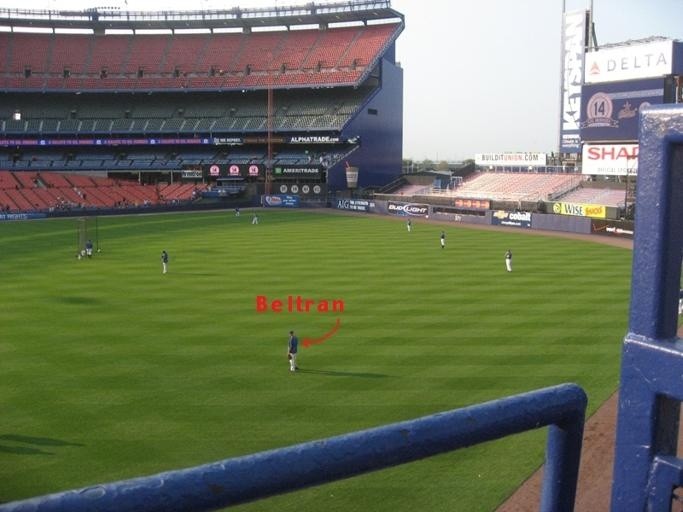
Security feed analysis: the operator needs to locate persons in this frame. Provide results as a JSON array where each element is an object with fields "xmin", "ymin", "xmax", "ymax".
[
  {"xmin": 678, "ymin": 287, "xmax": 683, "ymax": 315},
  {"xmin": 234, "ymin": 207, "xmax": 239, "ymax": 217},
  {"xmin": 439, "ymin": 230, "xmax": 446, "ymax": 248},
  {"xmin": 404, "ymin": 218, "xmax": 412, "ymax": 231},
  {"xmin": 286, "ymin": 328, "xmax": 300, "ymax": 373},
  {"xmin": 502, "ymin": 248, "xmax": 514, "ymax": 273},
  {"xmin": 161, "ymin": 249, "xmax": 169, "ymax": 274},
  {"xmin": 85, "ymin": 238, "xmax": 94, "ymax": 258},
  {"xmin": 251, "ymin": 213, "xmax": 259, "ymax": 225}
]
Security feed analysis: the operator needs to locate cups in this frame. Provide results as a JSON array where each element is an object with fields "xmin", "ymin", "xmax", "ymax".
[{"xmin": 345, "ymin": 166, "xmax": 360, "ymax": 190}]
